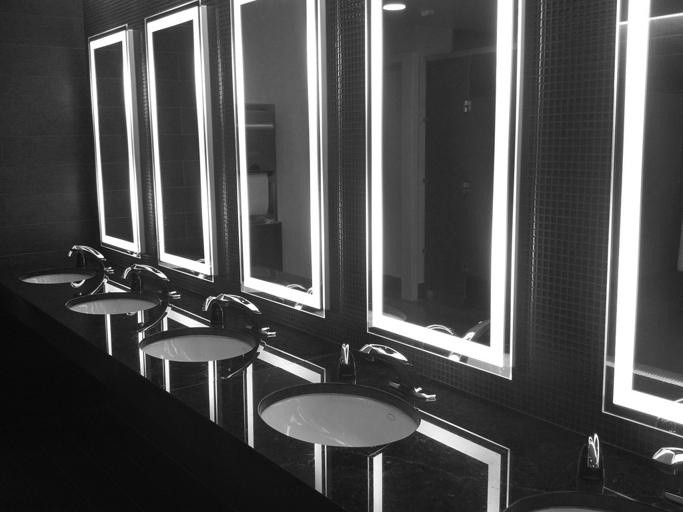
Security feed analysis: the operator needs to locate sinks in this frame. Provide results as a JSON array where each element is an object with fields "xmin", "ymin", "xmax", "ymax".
[
  {"xmin": 136, "ymin": 322, "xmax": 262, "ymax": 369},
  {"xmin": 16, "ymin": 267, "xmax": 97, "ymax": 286},
  {"xmin": 61, "ymin": 290, "xmax": 168, "ymax": 320},
  {"xmin": 504, "ymin": 489, "xmax": 660, "ymax": 512},
  {"xmin": 254, "ymin": 373, "xmax": 422, "ymax": 452}
]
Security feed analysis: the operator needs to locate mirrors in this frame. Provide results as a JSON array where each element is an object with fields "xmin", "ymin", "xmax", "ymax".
[
  {"xmin": 366, "ymin": 3, "xmax": 525, "ymax": 385},
  {"xmin": 146, "ymin": 0, "xmax": 214, "ymax": 284},
  {"xmin": 601, "ymin": 1, "xmax": 683, "ymax": 436},
  {"xmin": 88, "ymin": 21, "xmax": 142, "ymax": 260},
  {"xmin": 233, "ymin": 1, "xmax": 325, "ymax": 312}
]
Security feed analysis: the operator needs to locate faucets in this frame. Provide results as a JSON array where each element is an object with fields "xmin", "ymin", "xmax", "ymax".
[
  {"xmin": 65, "ymin": 243, "xmax": 107, "ymax": 281},
  {"xmin": 460, "ymin": 318, "xmax": 487, "ymax": 342},
  {"xmin": 119, "ymin": 260, "xmax": 172, "ymax": 297},
  {"xmin": 356, "ymin": 340, "xmax": 436, "ymax": 394},
  {"xmin": 650, "ymin": 445, "xmax": 683, "ymax": 510},
  {"xmin": 287, "ymin": 282, "xmax": 313, "ymax": 294},
  {"xmin": 202, "ymin": 292, "xmax": 263, "ymax": 329}
]
